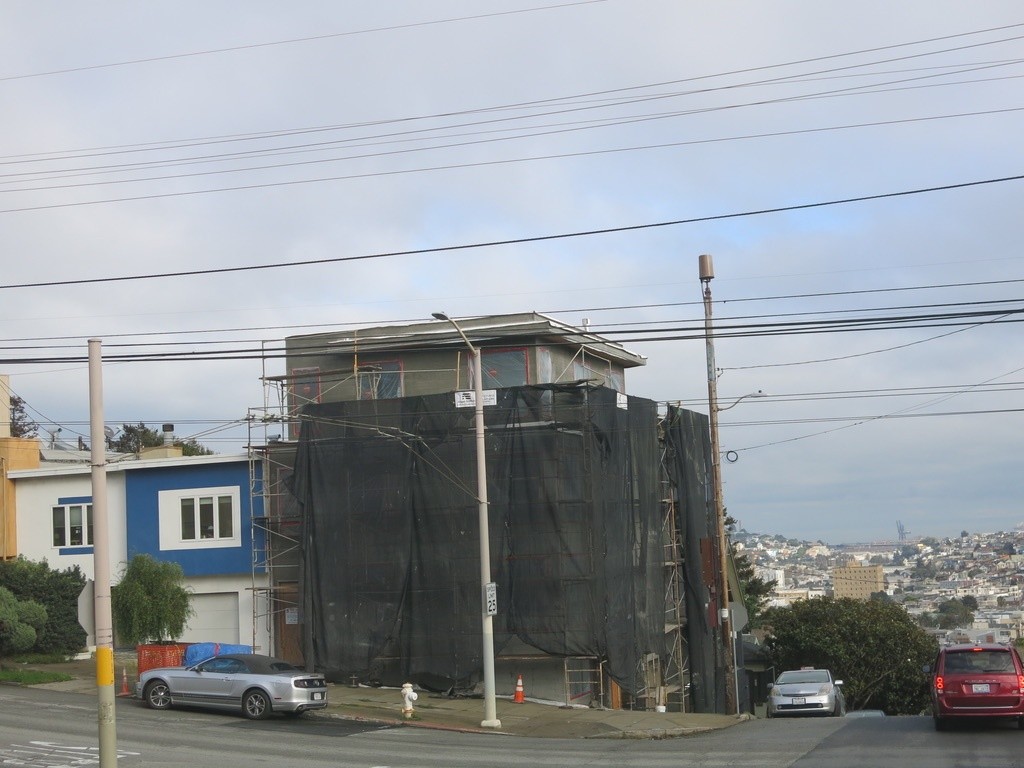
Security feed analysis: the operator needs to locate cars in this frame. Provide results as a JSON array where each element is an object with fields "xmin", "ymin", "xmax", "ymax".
[
  {"xmin": 845, "ymin": 711, "xmax": 885, "ymax": 717},
  {"xmin": 136, "ymin": 653, "xmax": 327, "ymax": 721},
  {"xmin": 766, "ymin": 669, "xmax": 844, "ymax": 717}
]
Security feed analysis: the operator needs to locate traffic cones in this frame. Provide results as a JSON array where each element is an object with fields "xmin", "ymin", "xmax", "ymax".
[
  {"xmin": 512, "ymin": 675, "xmax": 525, "ymax": 703},
  {"xmin": 116, "ymin": 667, "xmax": 130, "ymax": 696}
]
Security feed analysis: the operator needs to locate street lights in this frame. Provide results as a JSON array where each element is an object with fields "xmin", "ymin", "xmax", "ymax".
[
  {"xmin": 430, "ymin": 312, "xmax": 501, "ymax": 729},
  {"xmin": 708, "ymin": 392, "xmax": 767, "ymax": 715}
]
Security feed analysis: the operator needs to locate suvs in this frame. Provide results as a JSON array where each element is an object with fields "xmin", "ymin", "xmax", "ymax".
[{"xmin": 922, "ymin": 643, "xmax": 1024, "ymax": 732}]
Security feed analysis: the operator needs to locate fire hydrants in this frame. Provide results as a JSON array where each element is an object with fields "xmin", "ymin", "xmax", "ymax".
[{"xmin": 401, "ymin": 683, "xmax": 418, "ymax": 721}]
